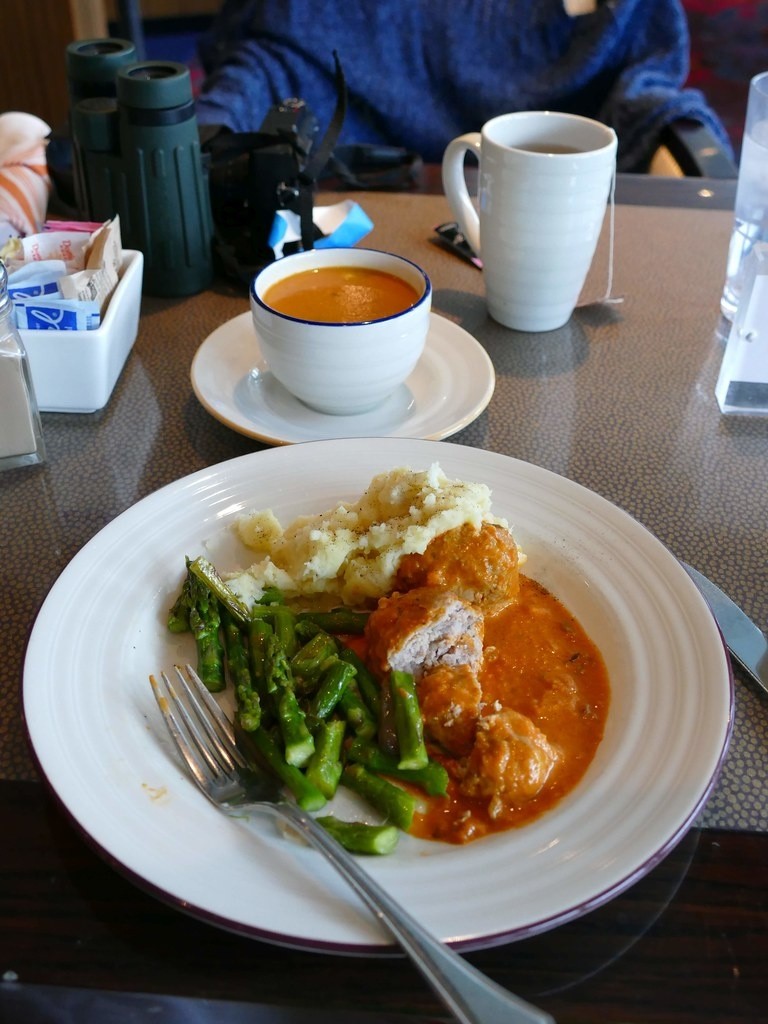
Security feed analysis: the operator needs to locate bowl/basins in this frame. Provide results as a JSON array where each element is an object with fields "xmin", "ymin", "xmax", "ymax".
[{"xmin": 7, "ymin": 249, "xmax": 145, "ymax": 418}]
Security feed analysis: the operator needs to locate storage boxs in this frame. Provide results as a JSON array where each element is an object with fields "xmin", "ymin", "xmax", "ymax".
[{"xmin": 17, "ymin": 250, "xmax": 144, "ymax": 413}]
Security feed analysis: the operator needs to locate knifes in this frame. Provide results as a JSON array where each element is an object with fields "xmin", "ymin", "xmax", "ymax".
[{"xmin": 673, "ymin": 554, "xmax": 768, "ymax": 693}]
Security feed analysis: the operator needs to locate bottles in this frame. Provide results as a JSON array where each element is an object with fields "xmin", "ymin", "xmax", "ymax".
[{"xmin": 0, "ymin": 260, "xmax": 47, "ymax": 470}]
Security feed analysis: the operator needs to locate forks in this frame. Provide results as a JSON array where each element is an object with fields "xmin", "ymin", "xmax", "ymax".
[{"xmin": 148, "ymin": 664, "xmax": 558, "ymax": 1024}]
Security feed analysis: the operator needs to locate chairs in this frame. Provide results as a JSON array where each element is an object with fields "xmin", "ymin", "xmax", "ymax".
[{"xmin": 195, "ymin": 0, "xmax": 738, "ymax": 179}]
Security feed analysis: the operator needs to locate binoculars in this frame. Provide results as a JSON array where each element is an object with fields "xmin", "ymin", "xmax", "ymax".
[{"xmin": 65, "ymin": 38, "xmax": 214, "ymax": 298}]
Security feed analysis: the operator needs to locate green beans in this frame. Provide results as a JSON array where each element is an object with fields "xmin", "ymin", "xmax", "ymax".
[{"xmin": 167, "ymin": 557, "xmax": 449, "ymax": 853}]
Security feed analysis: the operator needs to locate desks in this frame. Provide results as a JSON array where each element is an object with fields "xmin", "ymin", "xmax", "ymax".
[{"xmin": 0, "ymin": 159, "xmax": 768, "ymax": 1024}]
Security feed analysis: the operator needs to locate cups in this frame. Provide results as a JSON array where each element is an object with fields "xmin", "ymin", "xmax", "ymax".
[
  {"xmin": 247, "ymin": 247, "xmax": 433, "ymax": 416},
  {"xmin": 111, "ymin": 61, "xmax": 219, "ymax": 296},
  {"xmin": 442, "ymin": 111, "xmax": 617, "ymax": 334},
  {"xmin": 713, "ymin": 71, "xmax": 768, "ymax": 322},
  {"xmin": 64, "ymin": 37, "xmax": 140, "ymax": 225}
]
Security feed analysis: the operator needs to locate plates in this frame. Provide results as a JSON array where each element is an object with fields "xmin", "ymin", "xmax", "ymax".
[
  {"xmin": 21, "ymin": 439, "xmax": 738, "ymax": 962},
  {"xmin": 189, "ymin": 308, "xmax": 496, "ymax": 444}
]
